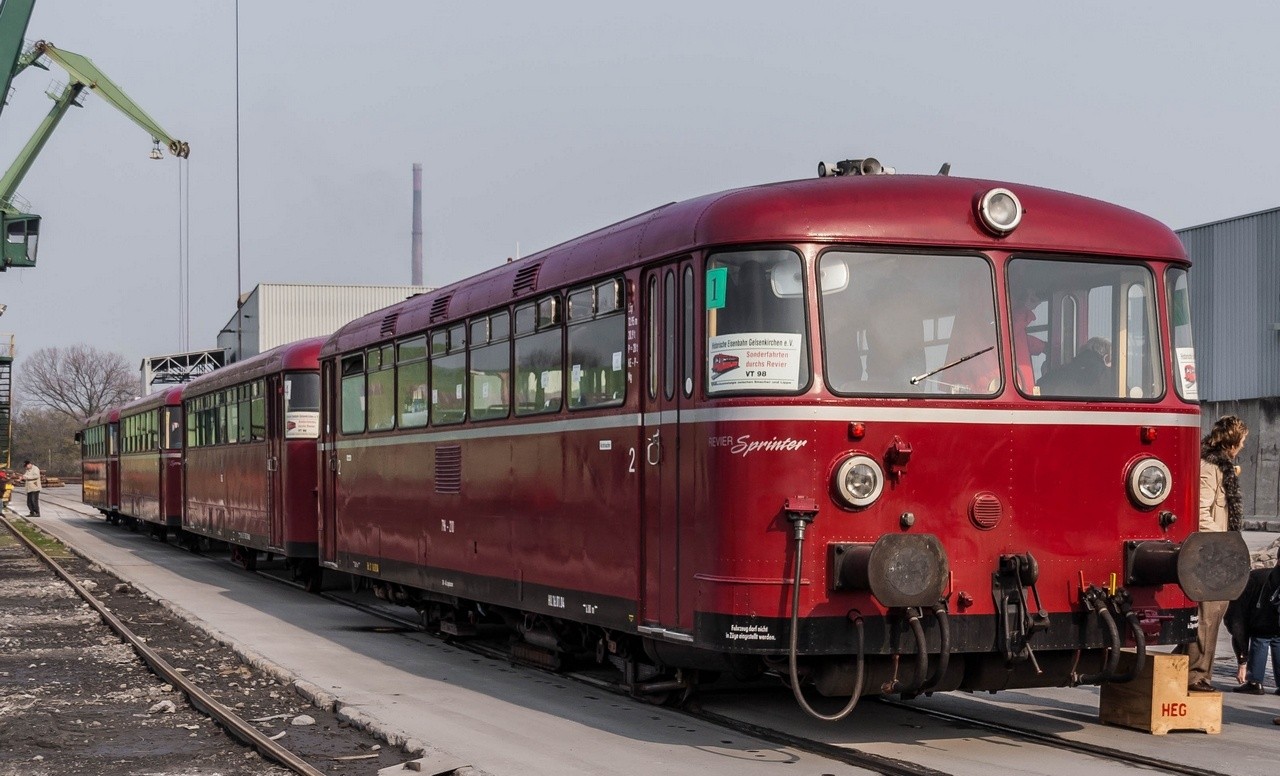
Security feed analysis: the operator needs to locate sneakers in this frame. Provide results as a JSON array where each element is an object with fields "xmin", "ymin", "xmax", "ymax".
[
  {"xmin": 1234, "ymin": 681, "xmax": 1265, "ymax": 695},
  {"xmin": 1275, "ymin": 688, "xmax": 1280, "ymax": 696}
]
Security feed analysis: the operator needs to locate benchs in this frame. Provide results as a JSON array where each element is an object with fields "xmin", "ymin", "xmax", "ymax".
[{"xmin": 483, "ymin": 391, "xmax": 621, "ymax": 417}]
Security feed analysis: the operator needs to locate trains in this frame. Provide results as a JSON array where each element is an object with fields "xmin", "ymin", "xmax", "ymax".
[{"xmin": 75, "ymin": 157, "xmax": 1253, "ymax": 725}]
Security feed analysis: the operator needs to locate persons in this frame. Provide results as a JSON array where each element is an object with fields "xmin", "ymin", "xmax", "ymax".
[
  {"xmin": 17, "ymin": 460, "xmax": 42, "ymax": 517},
  {"xmin": 1181, "ymin": 414, "xmax": 1279, "ymax": 695},
  {"xmin": 839, "ymin": 261, "xmax": 1131, "ymax": 401}
]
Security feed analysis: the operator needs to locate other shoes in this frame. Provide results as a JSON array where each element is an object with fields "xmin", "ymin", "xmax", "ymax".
[
  {"xmin": 27, "ymin": 513, "xmax": 34, "ymax": 516},
  {"xmin": 1273, "ymin": 717, "xmax": 1280, "ymax": 726},
  {"xmin": 33, "ymin": 513, "xmax": 40, "ymax": 517}
]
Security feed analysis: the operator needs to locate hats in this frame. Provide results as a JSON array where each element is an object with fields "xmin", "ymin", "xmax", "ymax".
[{"xmin": 23, "ymin": 461, "xmax": 30, "ymax": 468}]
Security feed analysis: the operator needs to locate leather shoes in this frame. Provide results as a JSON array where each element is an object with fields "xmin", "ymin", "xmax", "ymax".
[{"xmin": 1190, "ymin": 680, "xmax": 1215, "ymax": 692}]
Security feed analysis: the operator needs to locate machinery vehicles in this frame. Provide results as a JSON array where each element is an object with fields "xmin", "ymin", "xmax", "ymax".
[{"xmin": 0, "ymin": 1, "xmax": 190, "ymax": 300}]
